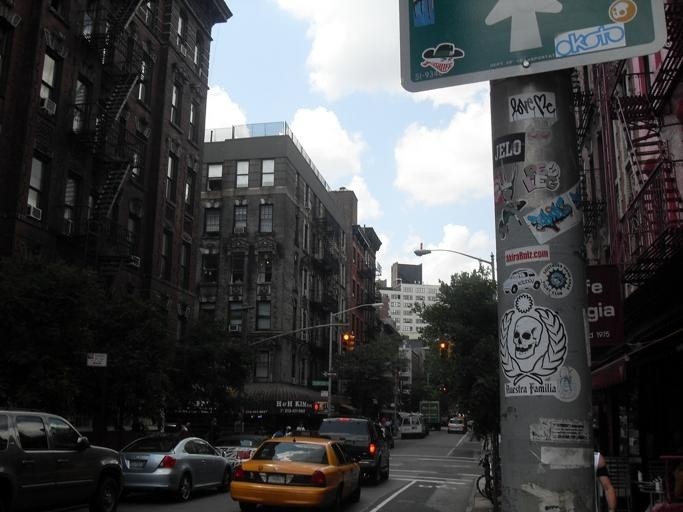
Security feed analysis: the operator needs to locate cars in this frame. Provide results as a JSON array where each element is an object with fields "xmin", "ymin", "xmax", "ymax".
[
  {"xmin": 318, "ymin": 418, "xmax": 389, "ymax": 486},
  {"xmin": 214, "ymin": 435, "xmax": 267, "ymax": 469},
  {"xmin": 120, "ymin": 436, "xmax": 232, "ymax": 500},
  {"xmin": 448, "ymin": 417, "xmax": 466, "ymax": 434},
  {"xmin": 230, "ymin": 436, "xmax": 360, "ymax": 512}
]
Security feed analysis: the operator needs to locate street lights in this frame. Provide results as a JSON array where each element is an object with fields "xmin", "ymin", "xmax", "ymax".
[
  {"xmin": 414, "ymin": 250, "xmax": 496, "ymax": 301},
  {"xmin": 328, "ymin": 303, "xmax": 384, "ymax": 418}
]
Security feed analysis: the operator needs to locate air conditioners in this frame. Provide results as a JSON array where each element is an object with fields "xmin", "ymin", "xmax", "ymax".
[
  {"xmin": 228, "ymin": 324, "xmax": 242, "ymax": 333},
  {"xmin": 234, "ymin": 225, "xmax": 246, "ymax": 235},
  {"xmin": 26, "ymin": 205, "xmax": 42, "ymax": 221},
  {"xmin": 129, "ymin": 255, "xmax": 141, "ymax": 268},
  {"xmin": 40, "ymin": 97, "xmax": 57, "ymax": 115}
]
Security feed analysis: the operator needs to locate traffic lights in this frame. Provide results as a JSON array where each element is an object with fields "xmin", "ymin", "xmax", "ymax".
[
  {"xmin": 342, "ymin": 329, "xmax": 357, "ymax": 352},
  {"xmin": 440, "ymin": 341, "xmax": 449, "ymax": 360}
]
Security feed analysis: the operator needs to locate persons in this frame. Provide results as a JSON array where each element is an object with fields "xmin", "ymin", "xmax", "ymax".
[
  {"xmin": 273, "ymin": 424, "xmax": 283, "ymax": 437},
  {"xmin": 284, "ymin": 425, "xmax": 293, "ymax": 436},
  {"xmin": 297, "ymin": 421, "xmax": 305, "ymax": 431},
  {"xmin": 177, "ymin": 422, "xmax": 193, "ymax": 431},
  {"xmin": 594, "ymin": 451, "xmax": 616, "ymax": 512}
]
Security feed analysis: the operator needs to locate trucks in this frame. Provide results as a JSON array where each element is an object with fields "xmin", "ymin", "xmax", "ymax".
[{"xmin": 419, "ymin": 400, "xmax": 441, "ymax": 431}]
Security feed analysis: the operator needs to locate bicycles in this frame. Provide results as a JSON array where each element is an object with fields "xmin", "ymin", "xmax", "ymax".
[{"xmin": 477, "ymin": 439, "xmax": 494, "ymax": 505}]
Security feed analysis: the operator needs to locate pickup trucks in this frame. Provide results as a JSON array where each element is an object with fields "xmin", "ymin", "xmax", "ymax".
[{"xmin": 399, "ymin": 415, "xmax": 426, "ymax": 439}]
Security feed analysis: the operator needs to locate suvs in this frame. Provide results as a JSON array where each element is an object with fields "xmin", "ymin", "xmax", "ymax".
[{"xmin": 0, "ymin": 411, "xmax": 124, "ymax": 512}]
